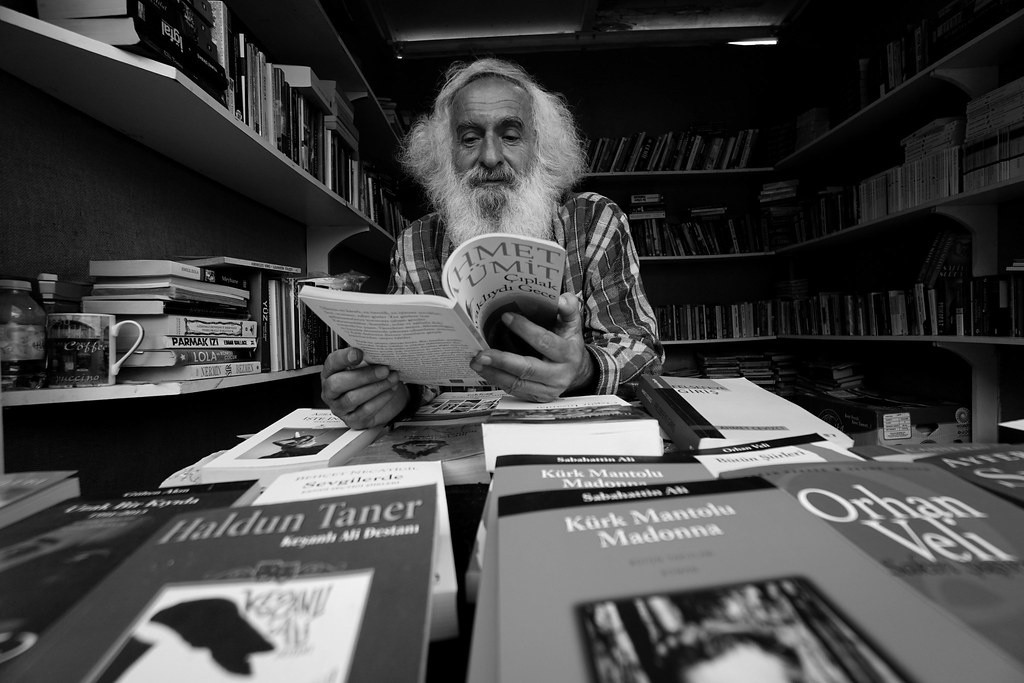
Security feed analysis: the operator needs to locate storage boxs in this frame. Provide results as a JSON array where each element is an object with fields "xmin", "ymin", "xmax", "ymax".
[{"xmin": 793, "ymin": 389, "xmax": 971, "ymax": 446}]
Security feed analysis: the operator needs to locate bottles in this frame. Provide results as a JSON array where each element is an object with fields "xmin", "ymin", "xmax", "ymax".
[{"xmin": 0, "ymin": 279, "xmax": 49, "ymax": 390}]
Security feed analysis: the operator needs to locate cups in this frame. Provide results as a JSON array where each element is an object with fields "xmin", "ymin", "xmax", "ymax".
[{"xmin": 43, "ymin": 311, "xmax": 144, "ymax": 390}]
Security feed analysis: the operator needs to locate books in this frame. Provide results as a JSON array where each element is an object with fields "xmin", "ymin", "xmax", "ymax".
[
  {"xmin": 0, "ymin": 377, "xmax": 1024, "ymax": 683},
  {"xmin": 650, "ymin": 298, "xmax": 772, "ymax": 341},
  {"xmin": 770, "ymin": 352, "xmax": 863, "ymax": 396},
  {"xmin": 770, "ymin": 232, "xmax": 1024, "ymax": 338},
  {"xmin": 299, "ymin": 230, "xmax": 569, "ymax": 386},
  {"xmin": 757, "ymin": 75, "xmax": 1024, "ymax": 242},
  {"xmin": 580, "ymin": 124, "xmax": 757, "ymax": 173},
  {"xmin": 623, "ymin": 192, "xmax": 765, "ymax": 255},
  {"xmin": 37, "ymin": 0, "xmax": 415, "ymax": 243},
  {"xmin": 35, "ymin": 254, "xmax": 365, "ymax": 377},
  {"xmin": 661, "ymin": 348, "xmax": 777, "ymax": 385},
  {"xmin": 793, "ymin": 0, "xmax": 1024, "ymax": 151}
]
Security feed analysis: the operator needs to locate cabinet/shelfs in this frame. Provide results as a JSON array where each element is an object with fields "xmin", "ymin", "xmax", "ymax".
[
  {"xmin": 579, "ymin": 168, "xmax": 778, "ymax": 345},
  {"xmin": 0, "ymin": 1, "xmax": 405, "ymax": 404},
  {"xmin": 777, "ymin": 7, "xmax": 1023, "ymax": 449}
]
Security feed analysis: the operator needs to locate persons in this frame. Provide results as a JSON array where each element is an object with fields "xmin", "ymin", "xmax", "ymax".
[{"xmin": 320, "ymin": 59, "xmax": 668, "ymax": 429}]
